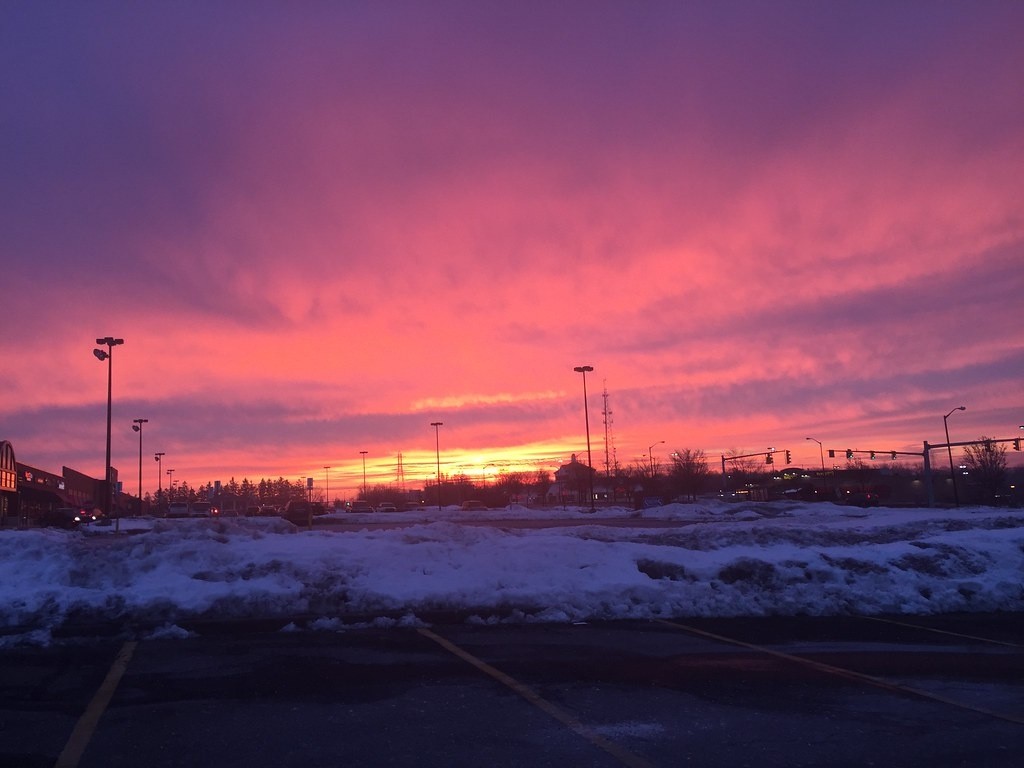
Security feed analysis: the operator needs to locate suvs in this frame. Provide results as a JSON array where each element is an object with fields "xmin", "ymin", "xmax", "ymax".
[{"xmin": 281, "ymin": 499, "xmax": 313, "ymax": 524}]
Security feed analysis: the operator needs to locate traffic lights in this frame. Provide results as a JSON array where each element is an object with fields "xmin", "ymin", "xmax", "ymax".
[
  {"xmin": 984, "ymin": 441, "xmax": 990, "ymax": 452},
  {"xmin": 786, "ymin": 450, "xmax": 791, "ymax": 464},
  {"xmin": 892, "ymin": 452, "xmax": 897, "ymax": 460},
  {"xmin": 871, "ymin": 451, "xmax": 875, "ymax": 460},
  {"xmin": 1013, "ymin": 440, "xmax": 1019, "ymax": 451},
  {"xmin": 768, "ymin": 453, "xmax": 773, "ymax": 464},
  {"xmin": 847, "ymin": 449, "xmax": 853, "ymax": 458}
]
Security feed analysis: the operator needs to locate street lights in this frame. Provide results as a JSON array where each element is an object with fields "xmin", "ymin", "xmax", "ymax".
[
  {"xmin": 649, "ymin": 441, "xmax": 665, "ymax": 477},
  {"xmin": 167, "ymin": 470, "xmax": 175, "ymax": 488},
  {"xmin": 360, "ymin": 452, "xmax": 368, "ymax": 493},
  {"xmin": 324, "ymin": 467, "xmax": 330, "ymax": 508},
  {"xmin": 574, "ymin": 366, "xmax": 594, "ymax": 509},
  {"xmin": 132, "ymin": 419, "xmax": 149, "ymax": 516},
  {"xmin": 806, "ymin": 438, "xmax": 825, "ymax": 485},
  {"xmin": 94, "ymin": 337, "xmax": 124, "ymax": 518},
  {"xmin": 943, "ymin": 406, "xmax": 966, "ymax": 507},
  {"xmin": 154, "ymin": 453, "xmax": 165, "ymax": 489},
  {"xmin": 432, "ymin": 423, "xmax": 443, "ymax": 511}
]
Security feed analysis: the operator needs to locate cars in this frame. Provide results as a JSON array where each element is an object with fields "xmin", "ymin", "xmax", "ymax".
[
  {"xmin": 39, "ymin": 507, "xmax": 105, "ymax": 531},
  {"xmin": 845, "ymin": 492, "xmax": 879, "ymax": 509},
  {"xmin": 166, "ymin": 502, "xmax": 397, "ymax": 518},
  {"xmin": 462, "ymin": 500, "xmax": 488, "ymax": 511}
]
